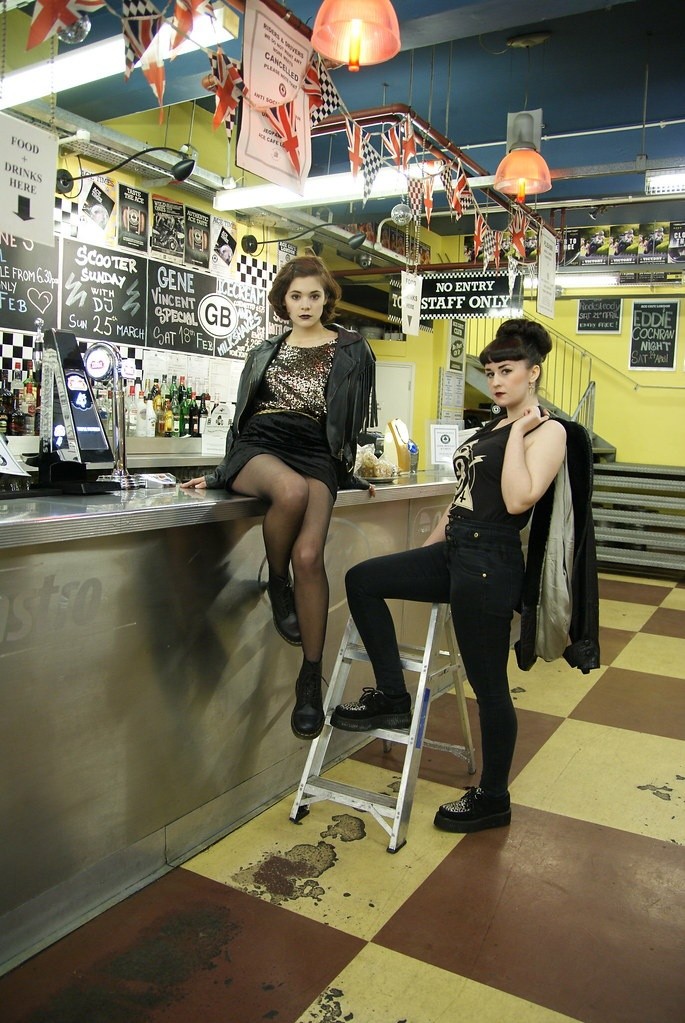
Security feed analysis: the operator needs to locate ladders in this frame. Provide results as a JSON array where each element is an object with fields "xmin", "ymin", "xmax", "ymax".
[{"xmin": 288, "ymin": 579, "xmax": 478, "ymax": 854}]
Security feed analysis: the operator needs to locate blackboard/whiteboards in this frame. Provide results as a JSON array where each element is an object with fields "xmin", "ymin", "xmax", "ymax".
[
  {"xmin": 1, "ymin": 231, "xmax": 59, "ymax": 335},
  {"xmin": 628, "ymin": 300, "xmax": 681, "ymax": 371},
  {"xmin": 216, "ymin": 276, "xmax": 267, "ymax": 361},
  {"xmin": 58, "ymin": 233, "xmax": 147, "ymax": 349},
  {"xmin": 268, "ymin": 301, "xmax": 293, "ymax": 339},
  {"xmin": 576, "ymin": 298, "xmax": 623, "ymax": 335},
  {"xmin": 148, "ymin": 256, "xmax": 218, "ymax": 359}
]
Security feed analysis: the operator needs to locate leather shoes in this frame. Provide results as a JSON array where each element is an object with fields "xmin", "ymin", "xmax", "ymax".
[
  {"xmin": 330, "ymin": 686, "xmax": 413, "ymax": 732},
  {"xmin": 434, "ymin": 785, "xmax": 512, "ymax": 832}
]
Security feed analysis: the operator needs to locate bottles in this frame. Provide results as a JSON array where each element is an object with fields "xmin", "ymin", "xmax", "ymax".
[
  {"xmin": 0, "ymin": 359, "xmax": 42, "ymax": 437},
  {"xmin": 116, "ymin": 369, "xmax": 220, "ymax": 438}
]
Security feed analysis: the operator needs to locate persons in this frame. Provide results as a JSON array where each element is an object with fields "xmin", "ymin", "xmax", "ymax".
[
  {"xmin": 179, "ymin": 253, "xmax": 379, "ymax": 739},
  {"xmin": 330, "ymin": 318, "xmax": 567, "ymax": 833}
]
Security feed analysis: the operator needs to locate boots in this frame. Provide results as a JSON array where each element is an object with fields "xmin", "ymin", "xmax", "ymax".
[
  {"xmin": 265, "ymin": 567, "xmax": 303, "ymax": 645},
  {"xmin": 289, "ymin": 655, "xmax": 331, "ymax": 740}
]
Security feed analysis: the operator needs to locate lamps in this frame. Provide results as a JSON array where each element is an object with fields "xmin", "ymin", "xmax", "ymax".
[
  {"xmin": 213, "ymin": 159, "xmax": 443, "ymax": 211},
  {"xmin": 311, "ymin": 0, "xmax": 402, "ymax": 72},
  {"xmin": 242, "ymin": 223, "xmax": 367, "ymax": 254},
  {"xmin": 0, "ymin": 0, "xmax": 240, "ymax": 111},
  {"xmin": 493, "ymin": 31, "xmax": 552, "ymax": 203},
  {"xmin": 588, "ymin": 204, "xmax": 606, "ymax": 221},
  {"xmin": 56, "ymin": 147, "xmax": 195, "ymax": 194},
  {"xmin": 374, "ymin": 204, "xmax": 412, "ymax": 251}
]
